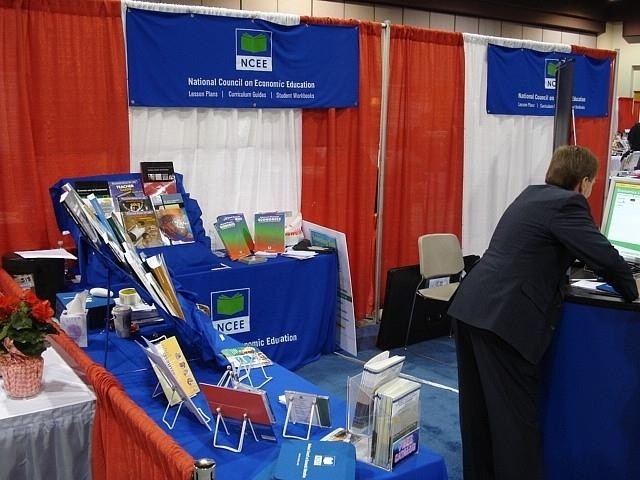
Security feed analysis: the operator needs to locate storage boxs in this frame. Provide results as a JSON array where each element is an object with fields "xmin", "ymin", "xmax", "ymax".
[{"xmin": 62, "ymin": 309, "xmax": 88, "ymax": 348}]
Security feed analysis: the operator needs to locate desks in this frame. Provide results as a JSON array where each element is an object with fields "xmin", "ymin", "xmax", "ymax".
[
  {"xmin": 40, "ymin": 281, "xmax": 446, "ymax": 480},
  {"xmin": 109, "ymin": 242, "xmax": 339, "ymax": 370}
]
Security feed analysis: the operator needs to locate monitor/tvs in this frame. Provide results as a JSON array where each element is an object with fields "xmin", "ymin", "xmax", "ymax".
[{"xmin": 600, "ymin": 175, "xmax": 640, "ymax": 266}]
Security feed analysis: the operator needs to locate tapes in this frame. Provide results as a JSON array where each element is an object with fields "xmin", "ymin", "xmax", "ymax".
[{"xmin": 118, "ymin": 287, "xmax": 140, "ymax": 304}]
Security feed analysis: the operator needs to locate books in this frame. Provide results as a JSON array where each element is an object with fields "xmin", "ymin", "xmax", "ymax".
[
  {"xmin": 351, "ymin": 350, "xmax": 421, "ymax": 470},
  {"xmin": 60, "ymin": 162, "xmax": 194, "ymax": 322},
  {"xmin": 213, "ymin": 211, "xmax": 285, "ymax": 261}
]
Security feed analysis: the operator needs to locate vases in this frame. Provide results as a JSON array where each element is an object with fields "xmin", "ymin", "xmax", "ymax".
[{"xmin": 1, "ymin": 355, "xmax": 44, "ymax": 401}]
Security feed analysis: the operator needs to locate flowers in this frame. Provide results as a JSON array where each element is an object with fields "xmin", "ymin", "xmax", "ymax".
[{"xmin": 0, "ymin": 289, "xmax": 61, "ymax": 355}]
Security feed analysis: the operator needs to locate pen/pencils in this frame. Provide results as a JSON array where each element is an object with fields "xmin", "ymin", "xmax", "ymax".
[{"xmin": 568, "ymin": 278, "xmax": 600, "ymax": 281}]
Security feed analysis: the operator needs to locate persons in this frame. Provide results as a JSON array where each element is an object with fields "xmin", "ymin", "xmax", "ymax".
[
  {"xmin": 445, "ymin": 146, "xmax": 639, "ymax": 480},
  {"xmin": 620, "ymin": 122, "xmax": 640, "ymax": 170}
]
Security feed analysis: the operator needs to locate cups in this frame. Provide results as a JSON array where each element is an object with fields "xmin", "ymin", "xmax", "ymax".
[{"xmin": 112, "ymin": 305, "xmax": 133, "ymax": 339}]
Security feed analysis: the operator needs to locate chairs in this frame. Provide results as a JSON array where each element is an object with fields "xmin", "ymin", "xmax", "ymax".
[{"xmin": 404, "ymin": 234, "xmax": 465, "ymax": 353}]
